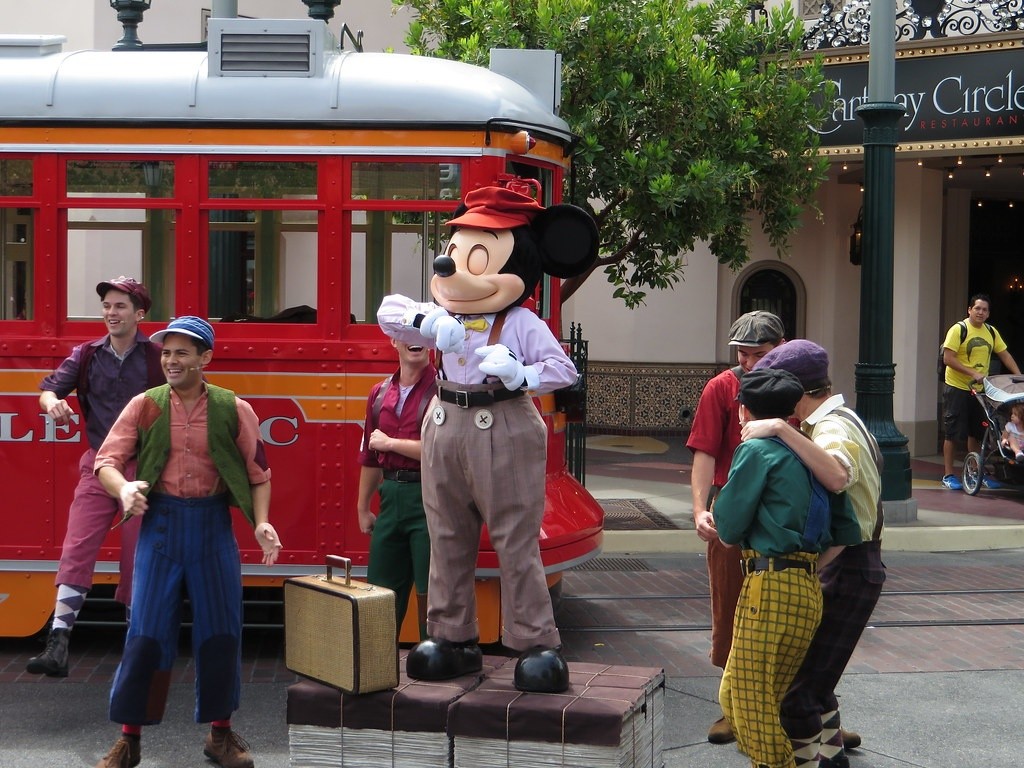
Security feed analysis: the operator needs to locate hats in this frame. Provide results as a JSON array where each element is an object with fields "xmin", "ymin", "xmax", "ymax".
[
  {"xmin": 728, "ymin": 311, "xmax": 783, "ymax": 346},
  {"xmin": 754, "ymin": 340, "xmax": 829, "ymax": 381},
  {"xmin": 148, "ymin": 315, "xmax": 215, "ymax": 350},
  {"xmin": 734, "ymin": 370, "xmax": 802, "ymax": 417},
  {"xmin": 96, "ymin": 276, "xmax": 151, "ymax": 313}
]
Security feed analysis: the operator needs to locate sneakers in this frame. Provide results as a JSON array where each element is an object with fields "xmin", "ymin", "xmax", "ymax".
[
  {"xmin": 95, "ymin": 736, "xmax": 140, "ymax": 768},
  {"xmin": 971, "ymin": 476, "xmax": 1001, "ymax": 489},
  {"xmin": 942, "ymin": 477, "xmax": 962, "ymax": 490},
  {"xmin": 204, "ymin": 729, "xmax": 254, "ymax": 768}
]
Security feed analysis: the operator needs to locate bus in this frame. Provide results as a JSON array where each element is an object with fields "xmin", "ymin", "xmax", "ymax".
[{"xmin": 0, "ymin": 0, "xmax": 607, "ymax": 643}]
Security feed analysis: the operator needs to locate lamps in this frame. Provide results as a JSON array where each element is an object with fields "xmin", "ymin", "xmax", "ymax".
[{"xmin": 850, "ymin": 206, "xmax": 864, "ymax": 265}]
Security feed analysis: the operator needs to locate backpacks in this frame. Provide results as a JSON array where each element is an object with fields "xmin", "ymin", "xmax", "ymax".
[{"xmin": 937, "ymin": 320, "xmax": 994, "ymax": 379}]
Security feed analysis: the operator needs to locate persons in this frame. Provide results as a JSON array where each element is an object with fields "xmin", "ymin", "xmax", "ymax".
[
  {"xmin": 1001, "ymin": 402, "xmax": 1024, "ymax": 460},
  {"xmin": 357, "ymin": 337, "xmax": 438, "ymax": 643},
  {"xmin": 713, "ymin": 366, "xmax": 863, "ymax": 768},
  {"xmin": 941, "ymin": 295, "xmax": 1021, "ymax": 489},
  {"xmin": 686, "ymin": 311, "xmax": 861, "ymax": 748},
  {"xmin": 92, "ymin": 316, "xmax": 281, "ymax": 768},
  {"xmin": 740, "ymin": 340, "xmax": 887, "ymax": 768},
  {"xmin": 25, "ymin": 277, "xmax": 167, "ymax": 678}
]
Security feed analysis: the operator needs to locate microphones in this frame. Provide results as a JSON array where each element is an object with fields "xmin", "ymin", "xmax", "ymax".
[
  {"xmin": 120, "ymin": 320, "xmax": 127, "ymax": 324},
  {"xmin": 189, "ymin": 365, "xmax": 208, "ymax": 371}
]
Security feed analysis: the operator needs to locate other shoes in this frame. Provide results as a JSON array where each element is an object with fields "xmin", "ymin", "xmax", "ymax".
[
  {"xmin": 842, "ymin": 728, "xmax": 860, "ymax": 747},
  {"xmin": 708, "ymin": 716, "xmax": 736, "ymax": 742}
]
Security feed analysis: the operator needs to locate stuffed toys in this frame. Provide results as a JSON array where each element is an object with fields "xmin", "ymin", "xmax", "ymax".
[{"xmin": 375, "ymin": 187, "xmax": 600, "ymax": 694}]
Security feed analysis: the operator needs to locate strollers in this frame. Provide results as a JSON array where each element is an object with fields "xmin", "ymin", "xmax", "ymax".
[{"xmin": 961, "ymin": 374, "xmax": 1024, "ymax": 499}]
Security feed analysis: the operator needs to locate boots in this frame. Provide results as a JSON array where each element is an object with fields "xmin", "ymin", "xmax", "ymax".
[{"xmin": 27, "ymin": 630, "xmax": 69, "ymax": 678}]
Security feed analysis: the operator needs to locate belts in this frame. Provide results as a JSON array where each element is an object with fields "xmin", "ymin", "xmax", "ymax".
[
  {"xmin": 738, "ymin": 558, "xmax": 811, "ymax": 576},
  {"xmin": 436, "ymin": 385, "xmax": 526, "ymax": 408},
  {"xmin": 382, "ymin": 469, "xmax": 423, "ymax": 485}
]
